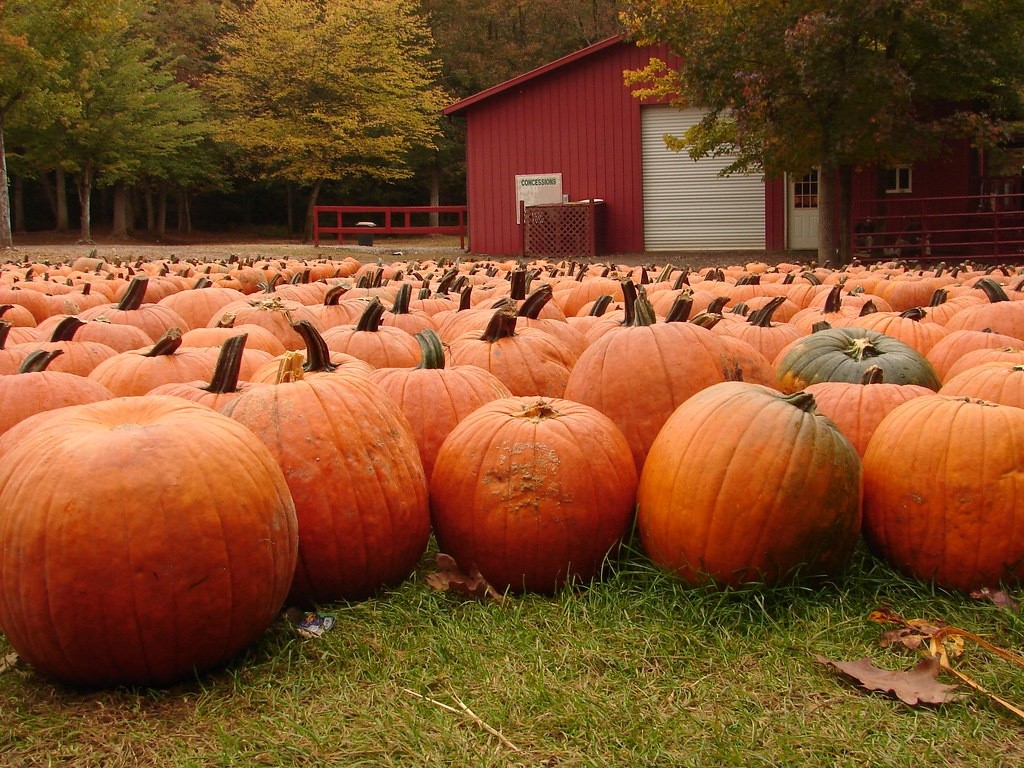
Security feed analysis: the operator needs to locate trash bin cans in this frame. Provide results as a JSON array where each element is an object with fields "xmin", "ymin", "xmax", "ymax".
[{"xmin": 356, "ymin": 221, "xmax": 377, "ymax": 247}]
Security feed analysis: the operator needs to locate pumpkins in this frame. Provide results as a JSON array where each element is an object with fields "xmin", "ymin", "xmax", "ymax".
[{"xmin": 0, "ymin": 249, "xmax": 1024, "ymax": 688}]
